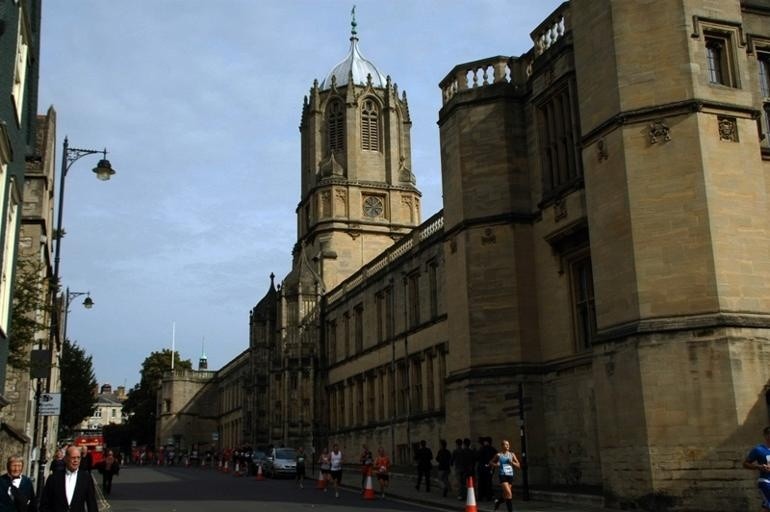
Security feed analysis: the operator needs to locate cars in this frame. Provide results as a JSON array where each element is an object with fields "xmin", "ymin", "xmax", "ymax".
[
  {"xmin": 57, "ymin": 438, "xmax": 74, "ymax": 447},
  {"xmin": 243, "ymin": 446, "xmax": 297, "ymax": 477}
]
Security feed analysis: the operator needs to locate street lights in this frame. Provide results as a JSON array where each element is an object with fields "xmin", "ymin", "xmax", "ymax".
[
  {"xmin": 55, "ymin": 285, "xmax": 95, "ymax": 453},
  {"xmin": 37, "ymin": 134, "xmax": 117, "ymax": 506}
]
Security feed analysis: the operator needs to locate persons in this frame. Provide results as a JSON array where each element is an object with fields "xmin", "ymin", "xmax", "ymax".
[
  {"xmin": 0, "ymin": 443, "xmax": 125, "ymax": 512},
  {"xmin": 128, "ymin": 443, "xmax": 213, "ymax": 469},
  {"xmin": 293, "ymin": 436, "xmax": 522, "ymax": 510}
]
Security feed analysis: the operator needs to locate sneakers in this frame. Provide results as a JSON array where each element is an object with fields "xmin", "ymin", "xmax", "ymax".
[{"xmin": 298, "ymin": 484, "xmax": 388, "ymax": 500}]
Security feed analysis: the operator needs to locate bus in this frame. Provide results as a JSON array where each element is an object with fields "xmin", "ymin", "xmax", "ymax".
[{"xmin": 76, "ymin": 433, "xmax": 105, "ymax": 467}]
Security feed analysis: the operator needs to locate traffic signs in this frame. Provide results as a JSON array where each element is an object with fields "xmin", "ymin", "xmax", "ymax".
[{"xmin": 501, "ymin": 391, "xmax": 533, "ymax": 418}]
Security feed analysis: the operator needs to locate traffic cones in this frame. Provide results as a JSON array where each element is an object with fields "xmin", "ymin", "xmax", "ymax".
[
  {"xmin": 217, "ymin": 457, "xmax": 223, "ymax": 471},
  {"xmin": 256, "ymin": 461, "xmax": 264, "ymax": 482},
  {"xmin": 185, "ymin": 458, "xmax": 189, "ymax": 468},
  {"xmin": 223, "ymin": 459, "xmax": 231, "ymax": 473},
  {"xmin": 463, "ymin": 475, "xmax": 477, "ymax": 512},
  {"xmin": 232, "ymin": 461, "xmax": 242, "ymax": 478},
  {"xmin": 315, "ymin": 470, "xmax": 324, "ymax": 489},
  {"xmin": 362, "ymin": 468, "xmax": 375, "ymax": 501}
]
